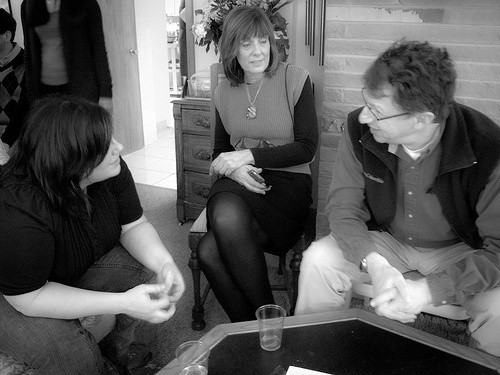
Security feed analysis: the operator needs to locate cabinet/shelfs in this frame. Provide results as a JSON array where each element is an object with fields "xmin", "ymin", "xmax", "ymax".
[{"xmin": 170, "ymin": 97, "xmax": 213, "ymax": 224}]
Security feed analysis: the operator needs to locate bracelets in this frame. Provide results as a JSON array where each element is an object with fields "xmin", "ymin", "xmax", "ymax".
[{"xmin": 360, "ymin": 259, "xmax": 367, "ymax": 273}]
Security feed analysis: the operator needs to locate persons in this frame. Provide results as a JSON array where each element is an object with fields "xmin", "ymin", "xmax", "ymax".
[
  {"xmin": 294, "ymin": 41, "xmax": 500, "ymax": 358},
  {"xmin": 0, "ymin": 97, "xmax": 186, "ymax": 375},
  {"xmin": 21, "ymin": 0, "xmax": 113, "ymax": 116},
  {"xmin": 196, "ymin": 5, "xmax": 319, "ymax": 322},
  {"xmin": 0, "ymin": 8, "xmax": 26, "ymax": 163}
]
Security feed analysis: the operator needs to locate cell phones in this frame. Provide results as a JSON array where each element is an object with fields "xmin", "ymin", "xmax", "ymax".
[{"xmin": 248, "ymin": 169, "xmax": 265, "ymax": 184}]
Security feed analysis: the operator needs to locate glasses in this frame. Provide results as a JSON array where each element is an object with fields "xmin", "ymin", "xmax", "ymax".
[{"xmin": 362, "ymin": 87, "xmax": 415, "ymax": 122}]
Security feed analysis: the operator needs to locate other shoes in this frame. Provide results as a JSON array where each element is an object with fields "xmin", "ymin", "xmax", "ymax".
[{"xmin": 100, "ymin": 336, "xmax": 152, "ymax": 369}]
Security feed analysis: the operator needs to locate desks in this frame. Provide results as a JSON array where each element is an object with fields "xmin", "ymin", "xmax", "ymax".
[{"xmin": 150, "ymin": 309, "xmax": 499, "ymax": 375}]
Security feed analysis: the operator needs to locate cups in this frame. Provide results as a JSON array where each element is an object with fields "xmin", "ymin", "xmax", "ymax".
[
  {"xmin": 174, "ymin": 341, "xmax": 210, "ymax": 375},
  {"xmin": 187, "ymin": 80, "xmax": 198, "ymax": 96},
  {"xmin": 255, "ymin": 303, "xmax": 286, "ymax": 351}
]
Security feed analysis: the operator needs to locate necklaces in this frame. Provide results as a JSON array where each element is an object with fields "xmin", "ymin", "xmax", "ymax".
[{"xmin": 244, "ymin": 77, "xmax": 266, "ymax": 119}]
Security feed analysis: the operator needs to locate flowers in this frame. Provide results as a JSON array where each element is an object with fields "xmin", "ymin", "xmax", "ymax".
[{"xmin": 195, "ymin": 0, "xmax": 295, "ymax": 64}]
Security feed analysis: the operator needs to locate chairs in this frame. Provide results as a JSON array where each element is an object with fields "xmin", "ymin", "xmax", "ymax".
[
  {"xmin": 351, "ymin": 271, "xmax": 471, "ymax": 322},
  {"xmin": 188, "ymin": 63, "xmax": 320, "ymax": 332}
]
